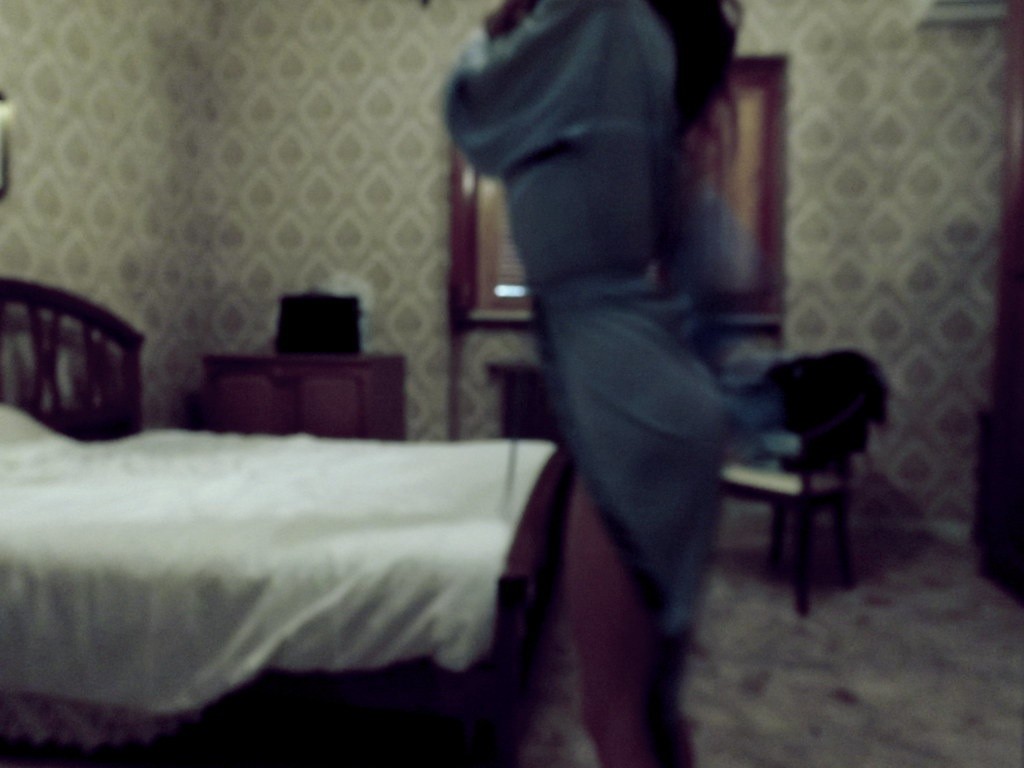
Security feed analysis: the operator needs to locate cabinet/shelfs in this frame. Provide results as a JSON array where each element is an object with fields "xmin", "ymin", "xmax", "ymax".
[{"xmin": 199, "ymin": 349, "xmax": 414, "ymax": 443}]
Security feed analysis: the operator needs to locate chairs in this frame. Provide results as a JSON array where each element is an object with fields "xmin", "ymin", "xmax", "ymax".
[{"xmin": 717, "ymin": 348, "xmax": 897, "ymax": 612}]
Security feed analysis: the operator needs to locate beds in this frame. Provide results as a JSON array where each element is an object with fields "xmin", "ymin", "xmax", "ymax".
[{"xmin": 1, "ymin": 420, "xmax": 563, "ymax": 767}]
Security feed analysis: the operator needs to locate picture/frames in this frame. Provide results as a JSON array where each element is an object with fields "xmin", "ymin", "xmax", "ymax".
[{"xmin": 441, "ymin": 52, "xmax": 793, "ymax": 337}]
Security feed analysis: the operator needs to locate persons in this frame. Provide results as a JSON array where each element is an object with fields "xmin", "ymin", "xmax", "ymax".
[{"xmin": 440, "ymin": 2, "xmax": 742, "ymax": 766}]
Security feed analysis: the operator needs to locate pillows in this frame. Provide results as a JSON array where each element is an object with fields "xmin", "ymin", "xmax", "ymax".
[{"xmin": 2, "ymin": 403, "xmax": 70, "ymax": 457}]
